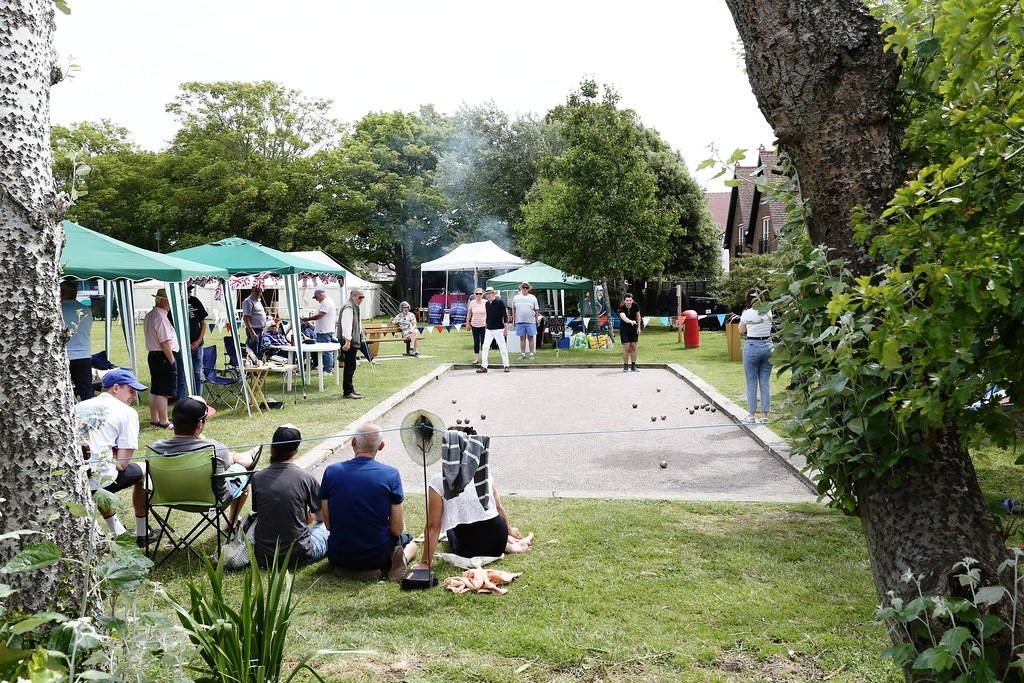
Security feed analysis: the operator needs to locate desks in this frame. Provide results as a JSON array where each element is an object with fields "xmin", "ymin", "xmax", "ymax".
[
  {"xmin": 117, "ymin": 308, "xmax": 151, "ymax": 326},
  {"xmin": 234, "ymin": 309, "xmax": 246, "ymax": 328},
  {"xmin": 236, "ymin": 368, "xmax": 272, "ymax": 417},
  {"xmin": 265, "ymin": 307, "xmax": 287, "ymax": 320},
  {"xmin": 251, "ymin": 365, "xmax": 297, "ymax": 409},
  {"xmin": 432, "ymin": 293, "xmax": 467, "ymax": 309},
  {"xmin": 282, "ymin": 343, "xmax": 341, "ymax": 392},
  {"xmin": 362, "ymin": 324, "xmax": 401, "ymax": 356}
]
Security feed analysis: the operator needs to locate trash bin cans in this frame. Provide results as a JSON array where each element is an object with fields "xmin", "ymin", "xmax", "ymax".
[
  {"xmin": 725, "ymin": 314, "xmax": 747, "ymax": 360},
  {"xmin": 682, "ymin": 309, "xmax": 700, "ymax": 349}
]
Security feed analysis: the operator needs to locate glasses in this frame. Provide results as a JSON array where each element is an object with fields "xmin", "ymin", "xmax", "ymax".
[
  {"xmin": 354, "ymin": 295, "xmax": 364, "ymax": 300},
  {"xmin": 270, "ymin": 325, "xmax": 276, "ymax": 327},
  {"xmin": 475, "ymin": 293, "xmax": 483, "ymax": 295},
  {"xmin": 522, "ymin": 287, "xmax": 529, "ymax": 290},
  {"xmin": 402, "ymin": 306, "xmax": 409, "ymax": 309},
  {"xmin": 585, "ymin": 296, "xmax": 590, "ymax": 298},
  {"xmin": 188, "ymin": 291, "xmax": 191, "ymax": 293}
]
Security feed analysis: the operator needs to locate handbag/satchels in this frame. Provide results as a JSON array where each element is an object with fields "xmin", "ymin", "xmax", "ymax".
[
  {"xmin": 213, "ymin": 513, "xmax": 258, "ymax": 569},
  {"xmin": 401, "ymin": 570, "xmax": 438, "ymax": 592}
]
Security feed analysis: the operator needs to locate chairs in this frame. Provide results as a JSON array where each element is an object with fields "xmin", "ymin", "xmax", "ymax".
[
  {"xmin": 427, "ymin": 302, "xmax": 443, "ymax": 324},
  {"xmin": 450, "ymin": 302, "xmax": 466, "ymax": 326},
  {"xmin": 145, "ymin": 445, "xmax": 262, "ymax": 575},
  {"xmin": 213, "ymin": 309, "xmax": 227, "ymax": 328},
  {"xmin": 224, "ymin": 335, "xmax": 248, "ymax": 379},
  {"xmin": 71, "ymin": 361, "xmax": 133, "ymax": 405},
  {"xmin": 202, "ymin": 345, "xmax": 247, "ymax": 411}
]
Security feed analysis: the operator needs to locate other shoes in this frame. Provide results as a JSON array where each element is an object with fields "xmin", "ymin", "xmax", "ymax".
[
  {"xmin": 318, "ymin": 371, "xmax": 332, "ymax": 376},
  {"xmin": 409, "ymin": 348, "xmax": 418, "ymax": 356},
  {"xmin": 334, "ymin": 545, "xmax": 405, "ymax": 582},
  {"xmin": 150, "ymin": 421, "xmax": 174, "ymax": 430},
  {"xmin": 342, "ymin": 392, "xmax": 362, "ymax": 399}
]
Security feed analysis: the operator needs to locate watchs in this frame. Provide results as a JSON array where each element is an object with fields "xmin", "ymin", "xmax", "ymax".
[{"xmin": 421, "ymin": 560, "xmax": 429, "ymax": 565}]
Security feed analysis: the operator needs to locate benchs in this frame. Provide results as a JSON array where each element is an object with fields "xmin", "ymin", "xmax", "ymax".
[{"xmin": 366, "ymin": 337, "xmax": 425, "ymax": 356}]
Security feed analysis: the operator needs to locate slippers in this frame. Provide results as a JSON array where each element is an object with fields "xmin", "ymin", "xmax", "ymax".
[
  {"xmin": 248, "ymin": 445, "xmax": 263, "ymax": 471},
  {"xmin": 225, "ymin": 517, "xmax": 242, "ymax": 532}
]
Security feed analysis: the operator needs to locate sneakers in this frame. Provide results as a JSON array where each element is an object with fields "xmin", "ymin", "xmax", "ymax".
[
  {"xmin": 111, "ymin": 525, "xmax": 165, "ymax": 548},
  {"xmin": 743, "ymin": 416, "xmax": 767, "ymax": 423},
  {"xmin": 623, "ymin": 363, "xmax": 639, "ymax": 372},
  {"xmin": 518, "ymin": 353, "xmax": 535, "ymax": 361},
  {"xmin": 473, "ymin": 359, "xmax": 479, "ymax": 363},
  {"xmin": 476, "ymin": 367, "xmax": 510, "ymax": 373}
]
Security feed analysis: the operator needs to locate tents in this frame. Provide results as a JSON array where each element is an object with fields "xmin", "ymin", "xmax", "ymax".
[
  {"xmin": 55, "ymin": 217, "xmax": 347, "ymax": 418},
  {"xmin": 418, "ymin": 240, "xmax": 530, "ymax": 310},
  {"xmin": 133, "ymin": 249, "xmax": 381, "ymax": 319},
  {"xmin": 486, "ymin": 260, "xmax": 600, "ymax": 350}
]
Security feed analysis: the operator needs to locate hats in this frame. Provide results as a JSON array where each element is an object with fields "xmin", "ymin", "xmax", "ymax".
[
  {"xmin": 484, "ymin": 287, "xmax": 494, "ymax": 293},
  {"xmin": 313, "ymin": 290, "xmax": 325, "ymax": 299},
  {"xmin": 519, "ymin": 281, "xmax": 532, "ymax": 290},
  {"xmin": 266, "ymin": 320, "xmax": 276, "ymax": 329},
  {"xmin": 400, "ymin": 409, "xmax": 446, "ymax": 465},
  {"xmin": 102, "ymin": 369, "xmax": 303, "ymax": 450},
  {"xmin": 151, "ymin": 285, "xmax": 195, "ymax": 300}
]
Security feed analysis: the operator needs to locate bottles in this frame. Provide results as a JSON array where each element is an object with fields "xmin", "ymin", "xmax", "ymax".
[
  {"xmin": 262, "ymin": 352, "xmax": 267, "ymax": 362},
  {"xmin": 291, "ymin": 335, "xmax": 295, "ymax": 346}
]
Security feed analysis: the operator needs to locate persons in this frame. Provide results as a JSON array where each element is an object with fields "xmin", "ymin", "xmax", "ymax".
[
  {"xmin": 250, "ymin": 426, "xmax": 330, "ymax": 570},
  {"xmin": 577, "ymin": 290, "xmax": 607, "ymax": 332},
  {"xmin": 319, "ymin": 419, "xmax": 418, "ymax": 583},
  {"xmin": 59, "ymin": 281, "xmax": 97, "ymax": 401},
  {"xmin": 142, "ymin": 282, "xmax": 208, "ymax": 430},
  {"xmin": 411, "ymin": 424, "xmax": 533, "ymax": 572},
  {"xmin": 465, "ymin": 285, "xmax": 512, "ymax": 373},
  {"xmin": 738, "ymin": 283, "xmax": 842, "ymax": 423},
  {"xmin": 512, "ymin": 280, "xmax": 540, "ymax": 360},
  {"xmin": 152, "ymin": 394, "xmax": 263, "ymax": 533},
  {"xmin": 392, "ymin": 301, "xmax": 420, "ymax": 358},
  {"xmin": 242, "ymin": 284, "xmax": 381, "ymax": 400},
  {"xmin": 618, "ymin": 293, "xmax": 641, "ymax": 372},
  {"xmin": 72, "ymin": 367, "xmax": 163, "ymax": 548}
]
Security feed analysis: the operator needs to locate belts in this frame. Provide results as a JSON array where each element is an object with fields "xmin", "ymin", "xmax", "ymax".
[{"xmin": 747, "ymin": 336, "xmax": 771, "ymax": 340}]
